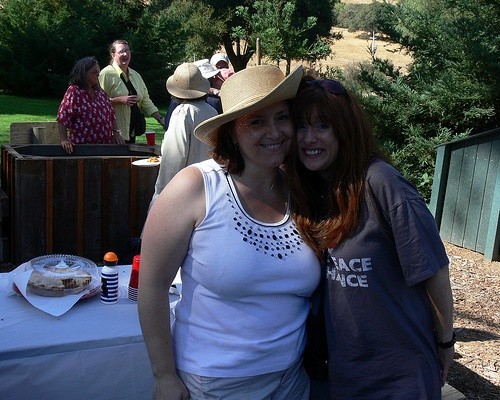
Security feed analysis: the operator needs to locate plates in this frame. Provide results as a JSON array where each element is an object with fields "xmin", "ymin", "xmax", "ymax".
[{"xmin": 132, "ymin": 158, "xmax": 160, "ymax": 167}]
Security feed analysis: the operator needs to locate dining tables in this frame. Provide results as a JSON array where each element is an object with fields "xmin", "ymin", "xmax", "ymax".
[{"xmin": 0, "ymin": 265, "xmax": 182, "ymax": 400}]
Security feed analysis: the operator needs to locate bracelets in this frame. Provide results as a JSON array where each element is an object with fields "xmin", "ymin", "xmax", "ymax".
[
  {"xmin": 113, "ymin": 129, "xmax": 120, "ymax": 133},
  {"xmin": 157, "ymin": 116, "xmax": 163, "ymax": 123},
  {"xmin": 436, "ymin": 330, "xmax": 456, "ymax": 349}
]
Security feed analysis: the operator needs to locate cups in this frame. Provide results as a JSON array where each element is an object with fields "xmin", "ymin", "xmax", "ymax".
[
  {"xmin": 146, "ymin": 132, "xmax": 156, "ymax": 147},
  {"xmin": 127, "ymin": 255, "xmax": 141, "ymax": 302}
]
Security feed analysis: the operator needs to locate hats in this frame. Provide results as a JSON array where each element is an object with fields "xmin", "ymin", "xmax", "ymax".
[
  {"xmin": 166, "ymin": 63, "xmax": 210, "ymax": 99},
  {"xmin": 193, "ymin": 59, "xmax": 220, "ymax": 78},
  {"xmin": 210, "ymin": 53, "xmax": 229, "ymax": 65},
  {"xmin": 194, "ymin": 65, "xmax": 303, "ymax": 146}
]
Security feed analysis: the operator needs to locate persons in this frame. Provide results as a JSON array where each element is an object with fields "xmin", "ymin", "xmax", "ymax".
[
  {"xmin": 165, "ymin": 58, "xmax": 223, "ymax": 129},
  {"xmin": 284, "ymin": 80, "xmax": 453, "ymax": 400},
  {"xmin": 148, "ymin": 63, "xmax": 218, "ymax": 214},
  {"xmin": 56, "ymin": 57, "xmax": 125, "ymax": 154},
  {"xmin": 137, "ymin": 65, "xmax": 321, "ymax": 400},
  {"xmin": 210, "ymin": 53, "xmax": 229, "ymax": 70},
  {"xmin": 97, "ymin": 40, "xmax": 165, "ymax": 144}
]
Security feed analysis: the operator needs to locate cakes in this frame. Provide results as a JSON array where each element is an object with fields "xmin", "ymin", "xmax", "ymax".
[{"xmin": 28, "ymin": 266, "xmax": 92, "ymax": 296}]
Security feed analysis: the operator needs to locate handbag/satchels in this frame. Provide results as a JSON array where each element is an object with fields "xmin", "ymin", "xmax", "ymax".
[{"xmin": 132, "ymin": 113, "xmax": 146, "ymax": 136}]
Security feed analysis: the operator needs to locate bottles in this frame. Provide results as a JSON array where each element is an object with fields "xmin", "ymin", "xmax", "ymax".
[{"xmin": 100, "ymin": 252, "xmax": 118, "ymax": 304}]
